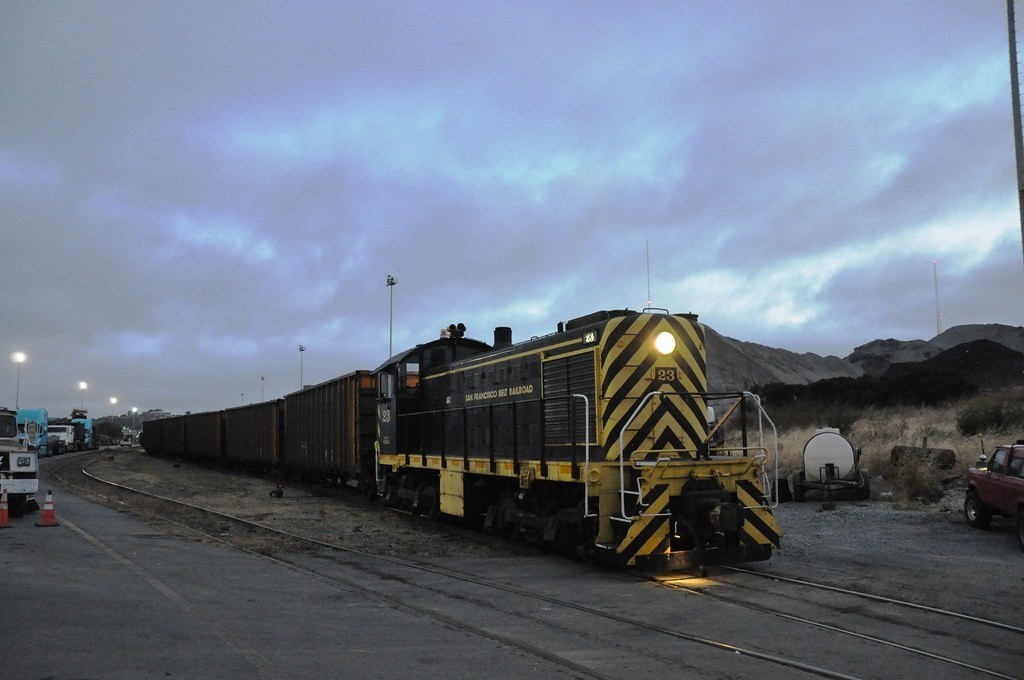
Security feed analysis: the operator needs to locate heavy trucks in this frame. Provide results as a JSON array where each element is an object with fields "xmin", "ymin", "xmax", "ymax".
[{"xmin": 0, "ymin": 407, "xmax": 100, "ymax": 519}]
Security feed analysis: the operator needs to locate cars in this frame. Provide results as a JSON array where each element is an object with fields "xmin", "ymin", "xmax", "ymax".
[{"xmin": 964, "ymin": 444, "xmax": 1024, "ymax": 530}]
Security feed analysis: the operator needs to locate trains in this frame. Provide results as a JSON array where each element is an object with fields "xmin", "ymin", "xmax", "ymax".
[{"xmin": 140, "ymin": 309, "xmax": 782, "ymax": 576}]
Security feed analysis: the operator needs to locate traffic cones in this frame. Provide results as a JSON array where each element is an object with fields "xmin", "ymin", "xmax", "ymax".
[
  {"xmin": 0, "ymin": 489, "xmax": 12, "ymax": 528},
  {"xmin": 35, "ymin": 490, "xmax": 60, "ymax": 527}
]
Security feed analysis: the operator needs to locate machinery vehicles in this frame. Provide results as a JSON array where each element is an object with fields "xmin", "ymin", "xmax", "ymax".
[{"xmin": 792, "ymin": 427, "xmax": 871, "ymax": 503}]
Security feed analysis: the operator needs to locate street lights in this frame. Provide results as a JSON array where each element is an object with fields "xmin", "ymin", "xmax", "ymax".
[
  {"xmin": 261, "ymin": 376, "xmax": 265, "ymax": 402},
  {"xmin": 16, "ymin": 355, "xmax": 23, "ymax": 408},
  {"xmin": 133, "ymin": 408, "xmax": 136, "ymax": 444},
  {"xmin": 386, "ymin": 274, "xmax": 397, "ymax": 358},
  {"xmin": 299, "ymin": 345, "xmax": 305, "ymax": 390},
  {"xmin": 111, "ymin": 398, "xmax": 115, "ymax": 423},
  {"xmin": 81, "ymin": 383, "xmax": 86, "ymax": 409}
]
